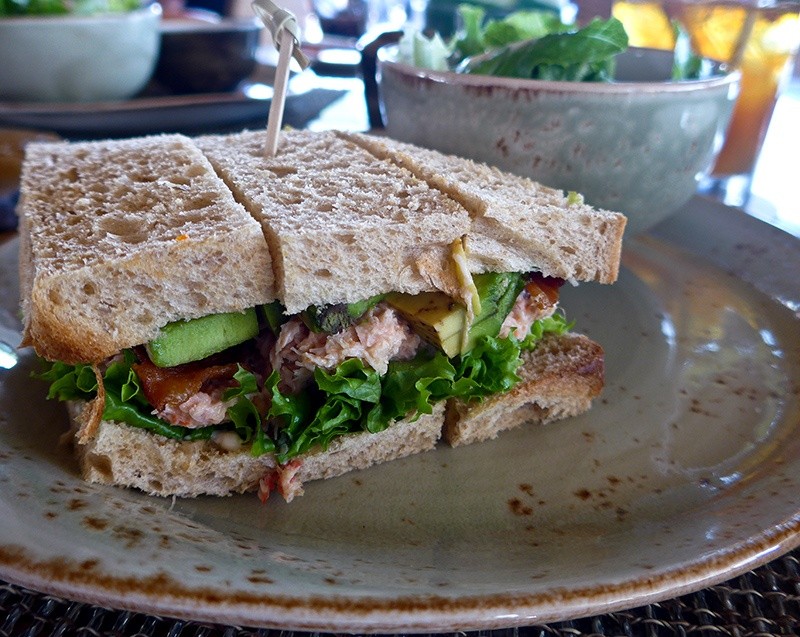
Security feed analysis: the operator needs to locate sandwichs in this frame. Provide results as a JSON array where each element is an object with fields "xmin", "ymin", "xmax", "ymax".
[{"xmin": 12, "ymin": 122, "xmax": 626, "ymax": 504}]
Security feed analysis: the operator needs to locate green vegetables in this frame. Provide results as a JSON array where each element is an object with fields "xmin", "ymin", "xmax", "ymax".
[
  {"xmin": 1, "ymin": 0, "xmax": 141, "ymax": 17},
  {"xmin": 394, "ymin": 1, "xmax": 707, "ymax": 84}
]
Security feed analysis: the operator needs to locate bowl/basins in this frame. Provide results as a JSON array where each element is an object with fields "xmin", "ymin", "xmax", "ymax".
[
  {"xmin": 375, "ymin": 38, "xmax": 743, "ymax": 238},
  {"xmin": 154, "ymin": 25, "xmax": 264, "ymax": 97},
  {"xmin": 1, "ymin": 2, "xmax": 164, "ymax": 104}
]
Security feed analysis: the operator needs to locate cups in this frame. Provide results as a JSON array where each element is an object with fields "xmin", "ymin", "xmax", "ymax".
[{"xmin": 607, "ymin": 0, "xmax": 799, "ymax": 208}]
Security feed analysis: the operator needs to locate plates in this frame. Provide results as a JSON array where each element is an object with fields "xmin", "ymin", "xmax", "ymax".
[
  {"xmin": 0, "ymin": 84, "xmax": 276, "ymax": 134},
  {"xmin": 2, "ymin": 189, "xmax": 799, "ymax": 636}
]
